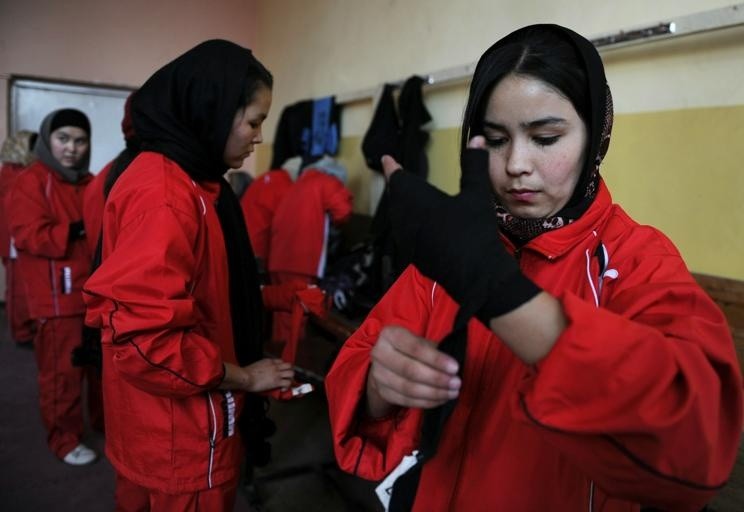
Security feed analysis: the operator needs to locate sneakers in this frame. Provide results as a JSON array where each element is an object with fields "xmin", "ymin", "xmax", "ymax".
[{"xmin": 63, "ymin": 444, "xmax": 97, "ymax": 466}]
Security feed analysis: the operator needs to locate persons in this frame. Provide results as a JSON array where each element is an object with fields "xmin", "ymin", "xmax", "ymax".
[
  {"xmin": 0, "ymin": 130, "xmax": 40, "ymax": 351},
  {"xmin": 79, "ymin": 38, "xmax": 327, "ymax": 511},
  {"xmin": 8, "ymin": 108, "xmax": 99, "ymax": 472},
  {"xmin": 326, "ymin": 22, "xmax": 743, "ymax": 512},
  {"xmin": 238, "ymin": 154, "xmax": 303, "ymax": 263},
  {"xmin": 268, "ymin": 153, "xmax": 357, "ymax": 403}
]
